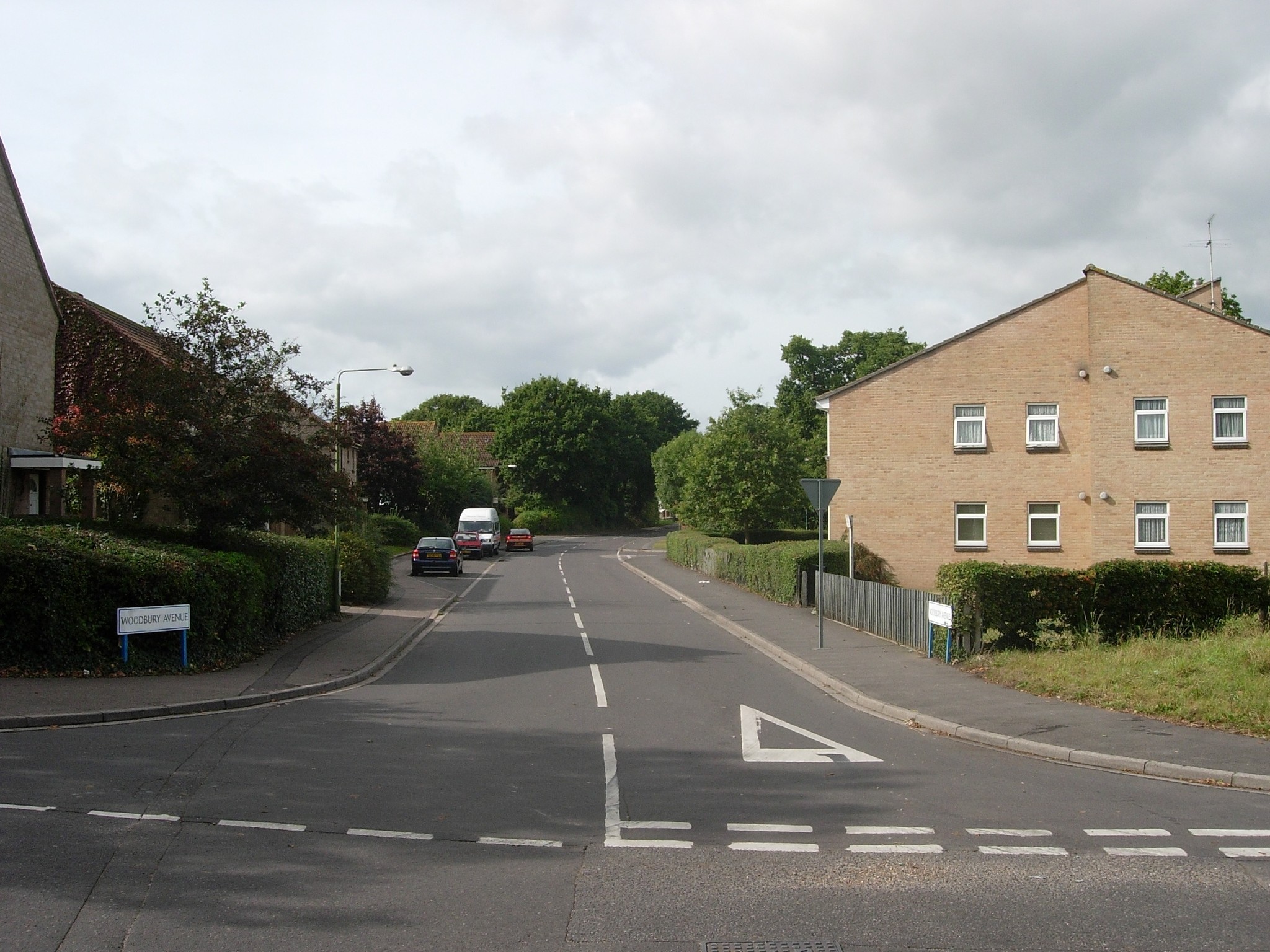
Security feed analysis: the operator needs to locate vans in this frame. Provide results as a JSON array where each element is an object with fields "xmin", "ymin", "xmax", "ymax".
[{"xmin": 458, "ymin": 507, "xmax": 502, "ymax": 556}]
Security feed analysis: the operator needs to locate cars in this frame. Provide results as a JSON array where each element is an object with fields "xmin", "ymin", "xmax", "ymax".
[
  {"xmin": 452, "ymin": 531, "xmax": 484, "ymax": 560},
  {"xmin": 505, "ymin": 528, "xmax": 535, "ymax": 552},
  {"xmin": 411, "ymin": 536, "xmax": 465, "ymax": 577}
]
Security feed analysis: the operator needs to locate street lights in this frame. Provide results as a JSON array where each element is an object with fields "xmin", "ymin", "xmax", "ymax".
[
  {"xmin": 490, "ymin": 464, "xmax": 518, "ymax": 510},
  {"xmin": 333, "ymin": 364, "xmax": 414, "ymax": 619}
]
